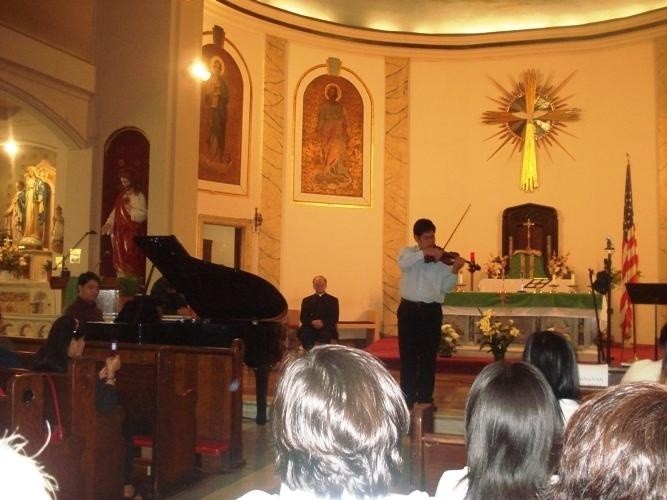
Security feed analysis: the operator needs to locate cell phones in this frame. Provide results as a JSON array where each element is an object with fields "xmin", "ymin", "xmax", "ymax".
[{"xmin": 110, "ymin": 339, "xmax": 119, "ymax": 357}]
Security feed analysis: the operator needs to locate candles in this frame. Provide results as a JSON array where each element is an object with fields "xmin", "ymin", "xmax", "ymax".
[{"xmin": 471, "ymin": 252, "xmax": 474, "ymax": 263}]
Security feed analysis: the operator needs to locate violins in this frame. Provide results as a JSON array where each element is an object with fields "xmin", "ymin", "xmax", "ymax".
[{"xmin": 424, "ymin": 246, "xmax": 481, "ymax": 272}]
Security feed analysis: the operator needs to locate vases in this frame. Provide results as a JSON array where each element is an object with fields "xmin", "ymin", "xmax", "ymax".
[{"xmin": 0, "ymin": 270, "xmax": 14, "ymax": 281}]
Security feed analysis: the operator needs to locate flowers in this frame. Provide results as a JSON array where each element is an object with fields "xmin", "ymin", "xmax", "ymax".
[
  {"xmin": 473, "ymin": 309, "xmax": 521, "ymax": 361},
  {"xmin": 546, "ymin": 250, "xmax": 570, "ymax": 279},
  {"xmin": 0, "ymin": 235, "xmax": 27, "ymax": 270},
  {"xmin": 487, "ymin": 254, "xmax": 510, "ymax": 279},
  {"xmin": 438, "ymin": 325, "xmax": 461, "ymax": 357},
  {"xmin": 547, "ymin": 326, "xmax": 579, "ymax": 358}
]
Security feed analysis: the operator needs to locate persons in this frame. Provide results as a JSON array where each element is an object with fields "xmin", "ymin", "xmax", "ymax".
[
  {"xmin": 397, "ymin": 219, "xmax": 464, "ymax": 410},
  {"xmin": 620, "ymin": 323, "xmax": 667, "ymax": 384},
  {"xmin": 545, "ymin": 380, "xmax": 666, "ymax": 500},
  {"xmin": 235, "ymin": 345, "xmax": 437, "ymax": 499},
  {"xmin": 202, "ymin": 61, "xmax": 228, "ymax": 166},
  {"xmin": 101, "ymin": 169, "xmax": 147, "ymax": 284},
  {"xmin": 3, "ymin": 180, "xmax": 64, "ymax": 253},
  {"xmin": 523, "ymin": 331, "xmax": 580, "ymax": 429},
  {"xmin": 435, "ymin": 359, "xmax": 566, "ymax": 500},
  {"xmin": 318, "ymin": 87, "xmax": 349, "ymax": 181},
  {"xmin": 0, "ymin": 271, "xmax": 199, "ymax": 500},
  {"xmin": 297, "ymin": 275, "xmax": 339, "ymax": 352}
]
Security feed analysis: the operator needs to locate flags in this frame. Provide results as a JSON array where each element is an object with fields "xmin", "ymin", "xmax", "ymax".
[{"xmin": 619, "ymin": 163, "xmax": 641, "ymax": 342}]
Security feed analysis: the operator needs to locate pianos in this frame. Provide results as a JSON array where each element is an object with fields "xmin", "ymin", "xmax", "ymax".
[{"xmin": 84, "ymin": 235, "xmax": 288, "ymax": 425}]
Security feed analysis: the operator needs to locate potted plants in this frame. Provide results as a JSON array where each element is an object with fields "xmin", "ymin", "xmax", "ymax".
[
  {"xmin": 42, "ymin": 260, "xmax": 52, "ymax": 281},
  {"xmin": 118, "ymin": 276, "xmax": 140, "ymax": 315}
]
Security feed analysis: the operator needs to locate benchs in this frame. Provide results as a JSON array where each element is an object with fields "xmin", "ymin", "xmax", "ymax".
[{"xmin": 0, "ymin": 336, "xmax": 467, "ymax": 500}]
